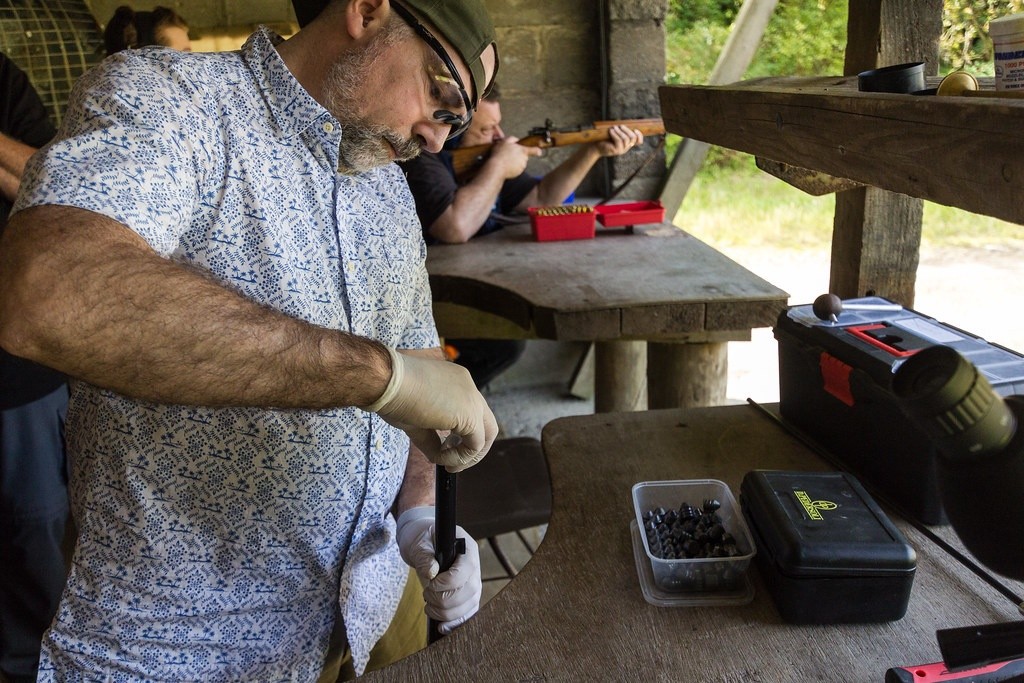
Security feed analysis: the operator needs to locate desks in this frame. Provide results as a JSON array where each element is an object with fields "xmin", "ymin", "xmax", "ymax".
[
  {"xmin": 347, "ymin": 400, "xmax": 1024, "ymax": 683},
  {"xmin": 424, "ymin": 196, "xmax": 790, "ymax": 413}
]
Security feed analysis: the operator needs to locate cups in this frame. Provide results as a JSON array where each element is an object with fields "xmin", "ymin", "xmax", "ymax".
[{"xmin": 858, "ymin": 61, "xmax": 927, "ymax": 93}]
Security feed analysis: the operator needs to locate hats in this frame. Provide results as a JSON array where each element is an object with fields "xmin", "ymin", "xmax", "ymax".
[{"xmin": 291, "ymin": 0, "xmax": 500, "ymax": 112}]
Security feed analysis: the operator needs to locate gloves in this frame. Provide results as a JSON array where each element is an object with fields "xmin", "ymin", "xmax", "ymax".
[
  {"xmin": 357, "ymin": 339, "xmax": 500, "ymax": 473},
  {"xmin": 396, "ymin": 506, "xmax": 482, "ymax": 635}
]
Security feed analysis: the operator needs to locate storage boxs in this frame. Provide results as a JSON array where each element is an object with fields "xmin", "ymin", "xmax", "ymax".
[
  {"xmin": 628, "ymin": 477, "xmax": 757, "ymax": 607},
  {"xmin": 740, "ymin": 470, "xmax": 918, "ymax": 626},
  {"xmin": 772, "ymin": 291, "xmax": 1024, "ymax": 527}
]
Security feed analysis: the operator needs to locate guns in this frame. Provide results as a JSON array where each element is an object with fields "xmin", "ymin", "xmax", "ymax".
[{"xmin": 447, "ymin": 116, "xmax": 665, "ymax": 177}]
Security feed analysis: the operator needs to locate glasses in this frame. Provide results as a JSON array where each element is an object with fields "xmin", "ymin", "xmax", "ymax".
[{"xmin": 389, "ymin": 0, "xmax": 473, "ymax": 141}]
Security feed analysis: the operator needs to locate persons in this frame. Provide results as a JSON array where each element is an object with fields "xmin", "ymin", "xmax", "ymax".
[
  {"xmin": 407, "ymin": 82, "xmax": 645, "ymax": 390},
  {"xmin": 0, "ymin": 1, "xmax": 500, "ymax": 682}
]
